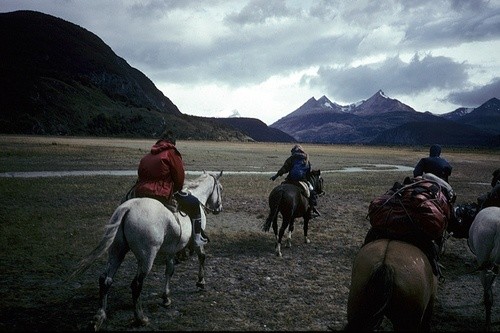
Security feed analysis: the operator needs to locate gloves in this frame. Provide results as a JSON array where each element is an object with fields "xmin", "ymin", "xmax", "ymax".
[{"xmin": 270, "ymin": 176, "xmax": 277, "ymax": 181}]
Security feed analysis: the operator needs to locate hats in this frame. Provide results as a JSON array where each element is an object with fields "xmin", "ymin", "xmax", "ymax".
[{"xmin": 429, "ymin": 144, "xmax": 442, "ymax": 153}]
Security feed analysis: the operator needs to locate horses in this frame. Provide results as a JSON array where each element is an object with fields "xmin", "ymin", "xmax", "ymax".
[
  {"xmin": 347, "ymin": 238, "xmax": 437, "ymax": 333},
  {"xmin": 64, "ymin": 168, "xmax": 224, "ymax": 332},
  {"xmin": 466, "ymin": 206, "xmax": 500, "ymax": 325},
  {"xmin": 261, "ymin": 169, "xmax": 325, "ymax": 257}
]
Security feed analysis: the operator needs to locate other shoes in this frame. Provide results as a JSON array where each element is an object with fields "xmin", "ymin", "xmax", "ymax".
[
  {"xmin": 195, "ymin": 232, "xmax": 208, "ymax": 246},
  {"xmin": 308, "ymin": 210, "xmax": 318, "ymax": 218}
]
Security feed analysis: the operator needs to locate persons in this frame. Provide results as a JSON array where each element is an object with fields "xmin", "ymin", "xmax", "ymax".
[
  {"xmin": 481, "ymin": 168, "xmax": 500, "ymax": 209},
  {"xmin": 413, "ymin": 145, "xmax": 462, "ymax": 228},
  {"xmin": 134, "ymin": 131, "xmax": 209, "ymax": 248},
  {"xmin": 269, "ymin": 144, "xmax": 319, "ymax": 219}
]
